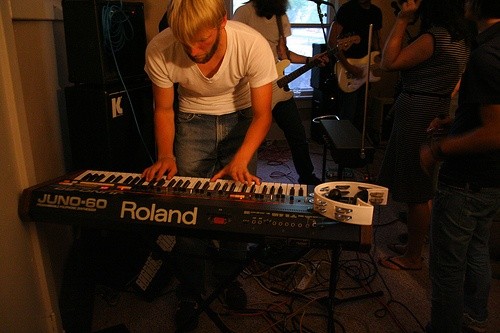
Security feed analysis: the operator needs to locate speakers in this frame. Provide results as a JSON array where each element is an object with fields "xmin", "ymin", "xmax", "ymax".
[
  {"xmin": 310, "ymin": 44, "xmax": 343, "ymax": 144},
  {"xmin": 60, "ymin": 0, "xmax": 178, "ymax": 292}
]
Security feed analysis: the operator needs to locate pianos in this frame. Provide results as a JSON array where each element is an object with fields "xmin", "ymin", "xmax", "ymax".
[{"xmin": 19, "ymin": 168, "xmax": 372, "ymax": 333}]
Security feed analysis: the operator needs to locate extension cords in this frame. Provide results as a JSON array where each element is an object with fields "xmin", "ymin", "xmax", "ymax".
[{"xmin": 297, "ymin": 263, "xmax": 321, "ymax": 292}]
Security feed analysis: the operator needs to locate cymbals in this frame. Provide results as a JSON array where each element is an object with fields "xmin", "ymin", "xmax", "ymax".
[{"xmin": 307, "ymin": 180, "xmax": 388, "ymax": 226}]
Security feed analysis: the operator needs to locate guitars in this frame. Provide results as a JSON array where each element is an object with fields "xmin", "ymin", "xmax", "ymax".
[
  {"xmin": 271, "ymin": 31, "xmax": 361, "ymax": 111},
  {"xmin": 334, "ymin": 51, "xmax": 382, "ymax": 93}
]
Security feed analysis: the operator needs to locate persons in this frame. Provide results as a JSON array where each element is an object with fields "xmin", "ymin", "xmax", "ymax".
[
  {"xmin": 326, "ymin": 0, "xmax": 384, "ymax": 124},
  {"xmin": 230, "ymin": 0, "xmax": 329, "ymax": 186},
  {"xmin": 141, "ymin": 0, "xmax": 278, "ymax": 333},
  {"xmin": 419, "ymin": 0, "xmax": 500, "ymax": 331},
  {"xmin": 379, "ymin": 0, "xmax": 472, "ymax": 271}
]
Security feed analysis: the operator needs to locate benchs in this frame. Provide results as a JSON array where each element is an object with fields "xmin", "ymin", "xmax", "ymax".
[{"xmin": 313, "ymin": 115, "xmax": 376, "ymax": 185}]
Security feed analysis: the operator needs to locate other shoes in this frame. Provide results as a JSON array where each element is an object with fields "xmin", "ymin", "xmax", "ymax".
[
  {"xmin": 463, "ymin": 312, "xmax": 489, "ymax": 333},
  {"xmin": 175, "ymin": 291, "xmax": 199, "ymax": 332},
  {"xmin": 398, "ymin": 233, "xmax": 407, "ymax": 244},
  {"xmin": 298, "ymin": 174, "xmax": 321, "ymax": 185},
  {"xmin": 399, "ymin": 212, "xmax": 407, "ymax": 223},
  {"xmin": 216, "ymin": 271, "xmax": 247, "ymax": 309}
]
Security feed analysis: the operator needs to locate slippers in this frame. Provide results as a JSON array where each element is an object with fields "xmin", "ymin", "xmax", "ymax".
[
  {"xmin": 379, "ymin": 254, "xmax": 419, "ymax": 271},
  {"xmin": 388, "ymin": 243, "xmax": 409, "ymax": 256}
]
